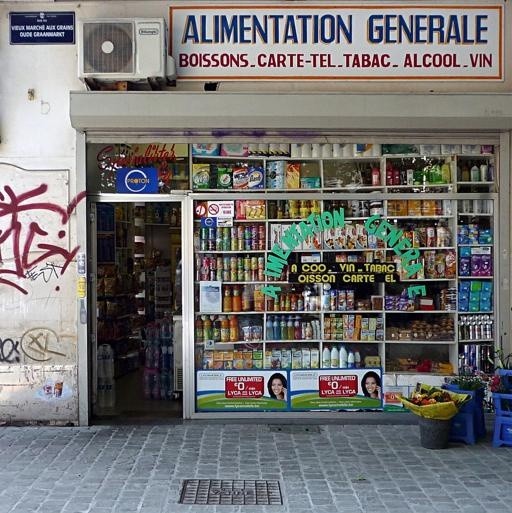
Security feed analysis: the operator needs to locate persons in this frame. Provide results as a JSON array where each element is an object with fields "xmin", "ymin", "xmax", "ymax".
[
  {"xmin": 268, "ymin": 373, "xmax": 287, "ymax": 400},
  {"xmin": 361, "ymin": 372, "xmax": 382, "ymax": 400}
]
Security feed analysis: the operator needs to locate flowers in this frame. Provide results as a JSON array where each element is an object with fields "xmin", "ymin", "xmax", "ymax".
[
  {"xmin": 486, "ymin": 344, "xmax": 511, "ymax": 370},
  {"xmin": 457, "ymin": 364, "xmax": 490, "ymax": 390},
  {"xmin": 394, "ymin": 382, "xmax": 472, "ymax": 420},
  {"xmin": 488, "ymin": 375, "xmax": 509, "ymax": 393}
]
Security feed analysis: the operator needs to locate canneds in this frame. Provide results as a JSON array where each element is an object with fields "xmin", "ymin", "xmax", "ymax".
[
  {"xmin": 359, "ymin": 200, "xmax": 369, "ymax": 215},
  {"xmin": 222, "ymin": 238, "xmax": 230, "ymax": 251},
  {"xmin": 245, "ymin": 239, "xmax": 251, "ymax": 250},
  {"xmin": 251, "ymin": 269, "xmax": 258, "ymax": 281},
  {"xmin": 209, "ymin": 257, "xmax": 217, "ymax": 270},
  {"xmin": 259, "ymin": 225, "xmax": 265, "ymax": 239},
  {"xmin": 223, "ymin": 270, "xmax": 230, "ymax": 282},
  {"xmin": 237, "ymin": 238, "xmax": 244, "ymax": 251},
  {"xmin": 257, "ymin": 257, "xmax": 265, "ymax": 268},
  {"xmin": 230, "ymin": 238, "xmax": 237, "ymax": 251},
  {"xmin": 237, "ymin": 257, "xmax": 244, "ymax": 269},
  {"xmin": 202, "ymin": 257, "xmax": 209, "ymax": 269},
  {"xmin": 222, "ymin": 228, "xmax": 230, "ymax": 239},
  {"xmin": 216, "ymin": 228, "xmax": 222, "ymax": 239},
  {"xmin": 268, "ymin": 202, "xmax": 276, "ymax": 219},
  {"xmin": 195, "ymin": 269, "xmax": 201, "ymax": 281},
  {"xmin": 200, "ymin": 228, "xmax": 208, "ymax": 239},
  {"xmin": 244, "ymin": 269, "xmax": 251, "ymax": 282},
  {"xmin": 216, "ymin": 270, "xmax": 223, "ymax": 282},
  {"xmin": 251, "ymin": 226, "xmax": 258, "ymax": 238},
  {"xmin": 245, "ymin": 226, "xmax": 251, "ymax": 239},
  {"xmin": 223, "ymin": 257, "xmax": 230, "ymax": 270},
  {"xmin": 230, "ymin": 269, "xmax": 237, "ymax": 282},
  {"xmin": 200, "ymin": 239, "xmax": 208, "ymax": 251},
  {"xmin": 457, "ymin": 343, "xmax": 494, "ymax": 375},
  {"xmin": 457, "ymin": 313, "xmax": 495, "ymax": 341},
  {"xmin": 237, "ymin": 269, "xmax": 244, "ymax": 281},
  {"xmin": 369, "ymin": 201, "xmax": 384, "ymax": 218},
  {"xmin": 244, "ymin": 257, "xmax": 250, "ymax": 269},
  {"xmin": 215, "ymin": 238, "xmax": 222, "ymax": 251},
  {"xmin": 258, "ymin": 239, "xmax": 266, "ymax": 250},
  {"xmin": 251, "ymin": 257, "xmax": 257, "ymax": 269},
  {"xmin": 210, "ymin": 270, "xmax": 216, "ymax": 282},
  {"xmin": 208, "ymin": 239, "xmax": 215, "ymax": 251},
  {"xmin": 238, "ymin": 226, "xmax": 244, "ymax": 239},
  {"xmin": 251, "ymin": 238, "xmax": 259, "ymax": 250},
  {"xmin": 461, "ymin": 166, "xmax": 470, "ymax": 181},
  {"xmin": 230, "ymin": 256, "xmax": 237, "ymax": 269},
  {"xmin": 208, "ymin": 228, "xmax": 216, "ymax": 239},
  {"xmin": 201, "ymin": 270, "xmax": 209, "ymax": 281},
  {"xmin": 217, "ymin": 257, "xmax": 223, "ymax": 270},
  {"xmin": 195, "ymin": 256, "xmax": 202, "ymax": 269},
  {"xmin": 230, "ymin": 226, "xmax": 238, "ymax": 238},
  {"xmin": 258, "ymin": 269, "xmax": 265, "ymax": 281}
]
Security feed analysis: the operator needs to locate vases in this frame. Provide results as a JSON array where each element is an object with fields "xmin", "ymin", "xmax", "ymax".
[
  {"xmin": 419, "ymin": 416, "xmax": 452, "ymax": 449},
  {"xmin": 498, "ymin": 370, "xmax": 512, "ymax": 384},
  {"xmin": 446, "ymin": 391, "xmax": 487, "ymax": 444},
  {"xmin": 491, "ymin": 393, "xmax": 512, "ymax": 449}
]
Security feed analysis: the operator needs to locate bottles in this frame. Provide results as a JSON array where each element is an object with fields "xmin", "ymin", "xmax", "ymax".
[
  {"xmin": 220, "ymin": 315, "xmax": 229, "ymax": 343},
  {"xmin": 372, "ymin": 167, "xmax": 380, "ymax": 187},
  {"xmin": 97, "ymin": 344, "xmax": 116, "ymax": 408},
  {"xmin": 143, "ymin": 311, "xmax": 174, "ymax": 398},
  {"xmin": 268, "ymin": 295, "xmax": 274, "ymax": 310},
  {"xmin": 196, "ymin": 347, "xmax": 263, "ymax": 368},
  {"xmin": 480, "ymin": 161, "xmax": 488, "ymax": 181},
  {"xmin": 291, "ymin": 294, "xmax": 297, "ymax": 311},
  {"xmin": 277, "ymin": 206, "xmax": 283, "ymax": 219},
  {"xmin": 291, "ymin": 349, "xmax": 301, "ymax": 369},
  {"xmin": 194, "ymin": 315, "xmax": 202, "ymax": 343},
  {"xmin": 202, "ymin": 315, "xmax": 211, "ymax": 342},
  {"xmin": 273, "ymin": 315, "xmax": 280, "ymax": 340},
  {"xmin": 330, "ymin": 346, "xmax": 339, "ymax": 369},
  {"xmin": 222, "ymin": 285, "xmax": 232, "ymax": 312},
  {"xmin": 265, "ymin": 350, "xmax": 271, "ymax": 368},
  {"xmin": 297, "ymin": 293, "xmax": 304, "ymax": 312},
  {"xmin": 322, "ymin": 346, "xmax": 330, "ymax": 369},
  {"xmin": 310, "ymin": 347, "xmax": 320, "ymax": 369},
  {"xmin": 354, "ymin": 350, "xmax": 361, "ymax": 368},
  {"xmin": 301, "ymin": 347, "xmax": 311, "ymax": 369},
  {"xmin": 280, "ymin": 292, "xmax": 285, "ymax": 311},
  {"xmin": 266, "ymin": 316, "xmax": 273, "ymax": 341},
  {"xmin": 287, "ymin": 315, "xmax": 294, "ymax": 340},
  {"xmin": 280, "ymin": 316, "xmax": 287, "ymax": 340},
  {"xmin": 347, "ymin": 351, "xmax": 354, "ymax": 369},
  {"xmin": 242, "ymin": 284, "xmax": 250, "ymax": 311},
  {"xmin": 282, "ymin": 349, "xmax": 292, "ymax": 369},
  {"xmin": 271, "ymin": 349, "xmax": 282, "ymax": 369},
  {"xmin": 232, "ymin": 284, "xmax": 241, "ymax": 311},
  {"xmin": 229, "ymin": 315, "xmax": 239, "ymax": 342},
  {"xmin": 168, "ymin": 207, "xmax": 181, "ymax": 227},
  {"xmin": 285, "ymin": 293, "xmax": 290, "ymax": 311},
  {"xmin": 154, "ymin": 265, "xmax": 172, "ymax": 311},
  {"xmin": 460, "ymin": 224, "xmax": 469, "ymax": 244},
  {"xmin": 212, "ymin": 316, "xmax": 220, "ymax": 342},
  {"xmin": 305, "ymin": 322, "xmax": 313, "ymax": 340},
  {"xmin": 274, "ymin": 294, "xmax": 280, "ymax": 311},
  {"xmin": 470, "ymin": 163, "xmax": 479, "ymax": 182},
  {"xmin": 294, "ymin": 315, "xmax": 302, "ymax": 340},
  {"xmin": 338, "ymin": 345, "xmax": 347, "ymax": 369}
]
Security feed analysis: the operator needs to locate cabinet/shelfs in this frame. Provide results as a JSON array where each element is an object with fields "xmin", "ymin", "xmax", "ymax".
[
  {"xmin": 97, "ymin": 203, "xmax": 135, "ymax": 274},
  {"xmin": 190, "ymin": 193, "xmax": 500, "ymax": 413},
  {"xmin": 86, "ymin": 133, "xmax": 500, "ymax": 195}
]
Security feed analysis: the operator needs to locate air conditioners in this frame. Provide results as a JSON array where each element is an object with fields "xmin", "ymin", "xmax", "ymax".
[{"xmin": 75, "ymin": 17, "xmax": 177, "ymax": 91}]
